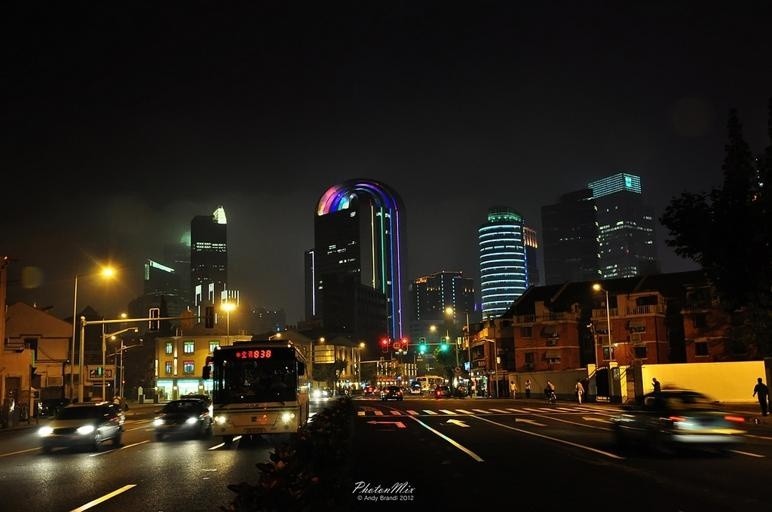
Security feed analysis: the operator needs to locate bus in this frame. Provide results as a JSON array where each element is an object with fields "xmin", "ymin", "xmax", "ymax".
[{"xmin": 212, "ymin": 339, "xmax": 312, "ymax": 445}]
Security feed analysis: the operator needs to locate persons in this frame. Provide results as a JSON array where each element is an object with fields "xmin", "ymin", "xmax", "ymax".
[
  {"xmin": 575, "ymin": 378, "xmax": 585, "ymax": 403},
  {"xmin": 651, "ymin": 377, "xmax": 660, "ymax": 394},
  {"xmin": 510, "ymin": 380, "xmax": 517, "ymax": 399},
  {"xmin": 524, "ymin": 378, "xmax": 532, "ymax": 399},
  {"xmin": 753, "ymin": 378, "xmax": 769, "ymax": 416},
  {"xmin": 546, "ymin": 381, "xmax": 556, "ymax": 402}
]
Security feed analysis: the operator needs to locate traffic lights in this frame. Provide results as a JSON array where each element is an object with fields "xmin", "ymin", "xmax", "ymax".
[
  {"xmin": 402, "ymin": 337, "xmax": 408, "ymax": 352},
  {"xmin": 441, "ymin": 337, "xmax": 448, "ymax": 350},
  {"xmin": 381, "ymin": 336, "xmax": 388, "ymax": 354},
  {"xmin": 418, "ymin": 337, "xmax": 426, "ymax": 352}
]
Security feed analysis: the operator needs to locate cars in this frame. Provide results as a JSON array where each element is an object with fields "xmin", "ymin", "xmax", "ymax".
[
  {"xmin": 312, "ymin": 386, "xmax": 330, "ymax": 397},
  {"xmin": 606, "ymin": 386, "xmax": 752, "ymax": 454},
  {"xmin": 349, "ymin": 374, "xmax": 469, "ymax": 402},
  {"xmin": 151, "ymin": 393, "xmax": 212, "ymax": 441},
  {"xmin": 39, "ymin": 399, "xmax": 128, "ymax": 451}
]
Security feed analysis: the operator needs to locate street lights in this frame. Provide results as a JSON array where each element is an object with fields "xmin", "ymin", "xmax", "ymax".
[
  {"xmin": 428, "ymin": 324, "xmax": 449, "ymax": 343},
  {"xmin": 351, "ymin": 341, "xmax": 367, "ymax": 388},
  {"xmin": 481, "ymin": 338, "xmax": 499, "ymax": 399},
  {"xmin": 66, "ymin": 259, "xmax": 144, "ymax": 404},
  {"xmin": 446, "ymin": 307, "xmax": 472, "ymax": 397},
  {"xmin": 592, "ymin": 284, "xmax": 613, "ymax": 361},
  {"xmin": 268, "ymin": 332, "xmax": 283, "ymax": 340},
  {"xmin": 220, "ymin": 300, "xmax": 239, "ymax": 347}
]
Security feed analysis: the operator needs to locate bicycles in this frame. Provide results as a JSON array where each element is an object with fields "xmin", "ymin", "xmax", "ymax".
[{"xmin": 543, "ymin": 388, "xmax": 559, "ymax": 406}]
